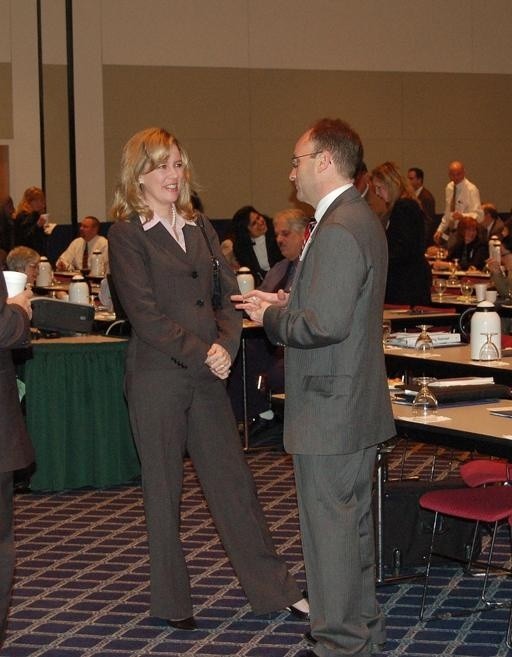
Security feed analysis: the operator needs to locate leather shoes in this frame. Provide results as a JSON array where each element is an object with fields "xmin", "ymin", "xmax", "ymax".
[{"xmin": 240, "ymin": 410, "xmax": 281, "ymax": 438}]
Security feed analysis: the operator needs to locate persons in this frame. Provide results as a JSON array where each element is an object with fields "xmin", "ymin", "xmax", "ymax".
[
  {"xmin": 108, "ymin": 127, "xmax": 314, "ymax": 629},
  {"xmin": 2, "ymin": 249, "xmax": 44, "ymax": 646},
  {"xmin": 191, "ymin": 190, "xmax": 310, "ymax": 440},
  {"xmin": 227, "ymin": 115, "xmax": 397, "ymax": 656},
  {"xmin": 1, "ymin": 185, "xmax": 113, "ymax": 310},
  {"xmin": 357, "ymin": 161, "xmax": 512, "ymax": 379}
]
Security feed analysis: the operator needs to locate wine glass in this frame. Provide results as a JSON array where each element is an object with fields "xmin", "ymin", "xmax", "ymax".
[
  {"xmin": 434, "ymin": 278, "xmax": 446, "ymax": 300},
  {"xmin": 480, "ymin": 332, "xmax": 499, "ymax": 361},
  {"xmin": 415, "ymin": 324, "xmax": 434, "ymax": 354},
  {"xmin": 447, "ymin": 265, "xmax": 459, "ymax": 284},
  {"xmin": 380, "ymin": 319, "xmax": 390, "ymax": 350},
  {"xmin": 460, "ymin": 280, "xmax": 472, "ymax": 301},
  {"xmin": 413, "ymin": 377, "xmax": 438, "ymax": 420}
]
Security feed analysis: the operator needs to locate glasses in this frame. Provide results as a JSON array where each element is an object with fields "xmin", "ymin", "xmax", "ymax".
[{"xmin": 291, "ymin": 152, "xmax": 321, "ymax": 168}]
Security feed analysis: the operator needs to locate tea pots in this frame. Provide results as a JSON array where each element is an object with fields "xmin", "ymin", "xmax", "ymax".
[
  {"xmin": 89, "ymin": 247, "xmax": 104, "ymax": 277},
  {"xmin": 37, "ymin": 256, "xmax": 53, "ymax": 287},
  {"xmin": 236, "ymin": 266, "xmax": 255, "ymax": 294},
  {"xmin": 458, "ymin": 301, "xmax": 501, "ymax": 361},
  {"xmin": 489, "ymin": 234, "xmax": 501, "ymax": 266},
  {"xmin": 69, "ymin": 274, "xmax": 92, "ymax": 303}
]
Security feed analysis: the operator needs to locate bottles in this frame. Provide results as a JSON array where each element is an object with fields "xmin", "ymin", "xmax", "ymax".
[{"xmin": 439, "ymin": 248, "xmax": 444, "ymax": 257}]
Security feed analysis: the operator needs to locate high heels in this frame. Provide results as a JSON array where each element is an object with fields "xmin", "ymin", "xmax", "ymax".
[
  {"xmin": 269, "ymin": 605, "xmax": 310, "ymax": 620},
  {"xmin": 166, "ymin": 617, "xmax": 198, "ymax": 630}
]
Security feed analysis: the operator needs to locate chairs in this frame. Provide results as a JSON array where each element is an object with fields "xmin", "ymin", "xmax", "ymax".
[{"xmin": 415, "ymin": 457, "xmax": 512, "ymax": 623}]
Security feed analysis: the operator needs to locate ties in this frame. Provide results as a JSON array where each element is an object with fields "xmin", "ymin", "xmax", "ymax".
[
  {"xmin": 295, "ymin": 217, "xmax": 317, "ymax": 256},
  {"xmin": 83, "ymin": 243, "xmax": 88, "ymax": 268}
]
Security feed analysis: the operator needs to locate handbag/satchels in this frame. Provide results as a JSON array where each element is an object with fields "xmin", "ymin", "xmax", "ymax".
[{"xmin": 198, "ymin": 216, "xmax": 222, "ymax": 309}]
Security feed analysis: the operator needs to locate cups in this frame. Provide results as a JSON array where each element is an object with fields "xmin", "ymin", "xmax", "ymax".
[
  {"xmin": 484, "ymin": 290, "xmax": 495, "ymax": 301},
  {"xmin": 3, "ymin": 270, "xmax": 28, "ymax": 297},
  {"xmin": 452, "ymin": 258, "xmax": 459, "ymax": 265},
  {"xmin": 475, "ymin": 284, "xmax": 486, "ymax": 299}
]
